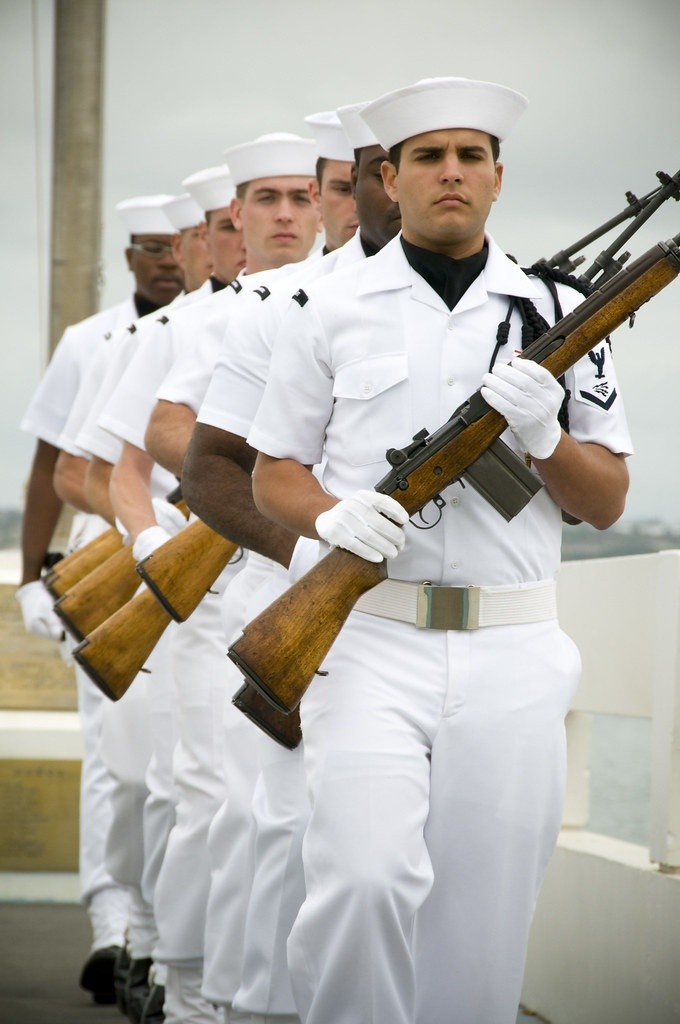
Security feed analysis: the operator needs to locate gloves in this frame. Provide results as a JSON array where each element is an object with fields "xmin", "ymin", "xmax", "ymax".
[
  {"xmin": 481, "ymin": 357, "xmax": 565, "ymax": 459},
  {"xmin": 286, "ymin": 535, "xmax": 320, "ymax": 583},
  {"xmin": 315, "ymin": 490, "xmax": 409, "ymax": 563},
  {"xmin": 15, "ymin": 580, "xmax": 63, "ymax": 640},
  {"xmin": 132, "ymin": 526, "xmax": 171, "ymax": 562},
  {"xmin": 115, "ymin": 497, "xmax": 189, "ymax": 545}
]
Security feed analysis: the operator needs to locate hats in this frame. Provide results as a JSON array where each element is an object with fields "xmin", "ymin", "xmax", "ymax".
[
  {"xmin": 117, "ymin": 194, "xmax": 178, "ymax": 235},
  {"xmin": 163, "ymin": 193, "xmax": 207, "ymax": 230},
  {"xmin": 223, "ymin": 131, "xmax": 318, "ymax": 186},
  {"xmin": 337, "ymin": 101, "xmax": 380, "ymax": 149},
  {"xmin": 360, "ymin": 77, "xmax": 529, "ymax": 153},
  {"xmin": 304, "ymin": 110, "xmax": 357, "ymax": 163},
  {"xmin": 183, "ymin": 165, "xmax": 237, "ymax": 212}
]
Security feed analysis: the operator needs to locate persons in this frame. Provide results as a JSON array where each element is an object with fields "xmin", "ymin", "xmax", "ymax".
[
  {"xmin": 144, "ymin": 112, "xmax": 357, "ymax": 1024},
  {"xmin": 15, "ymin": 193, "xmax": 212, "ymax": 1024},
  {"xmin": 178, "ymin": 97, "xmax": 403, "ymax": 1024},
  {"xmin": 247, "ymin": 78, "xmax": 634, "ymax": 1024},
  {"xmin": 96, "ymin": 166, "xmax": 246, "ymax": 1024}
]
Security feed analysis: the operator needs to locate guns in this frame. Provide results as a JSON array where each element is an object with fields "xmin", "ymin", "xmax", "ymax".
[
  {"xmin": 230, "ymin": 169, "xmax": 680, "ymax": 753},
  {"xmin": 132, "ymin": 184, "xmax": 665, "ymax": 625},
  {"xmin": 70, "ymin": 586, "xmax": 175, "ymax": 704},
  {"xmin": 40, "ymin": 526, "xmax": 127, "ymax": 601},
  {"xmin": 52, "ymin": 541, "xmax": 145, "ymax": 644},
  {"xmin": 224, "ymin": 230, "xmax": 680, "ymax": 717}
]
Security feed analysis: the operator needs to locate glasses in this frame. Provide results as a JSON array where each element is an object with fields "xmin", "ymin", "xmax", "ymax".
[{"xmin": 129, "ymin": 242, "xmax": 173, "ymax": 257}]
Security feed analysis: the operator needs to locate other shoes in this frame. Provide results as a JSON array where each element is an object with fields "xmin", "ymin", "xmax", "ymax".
[{"xmin": 79, "ymin": 944, "xmax": 166, "ymax": 1024}]
